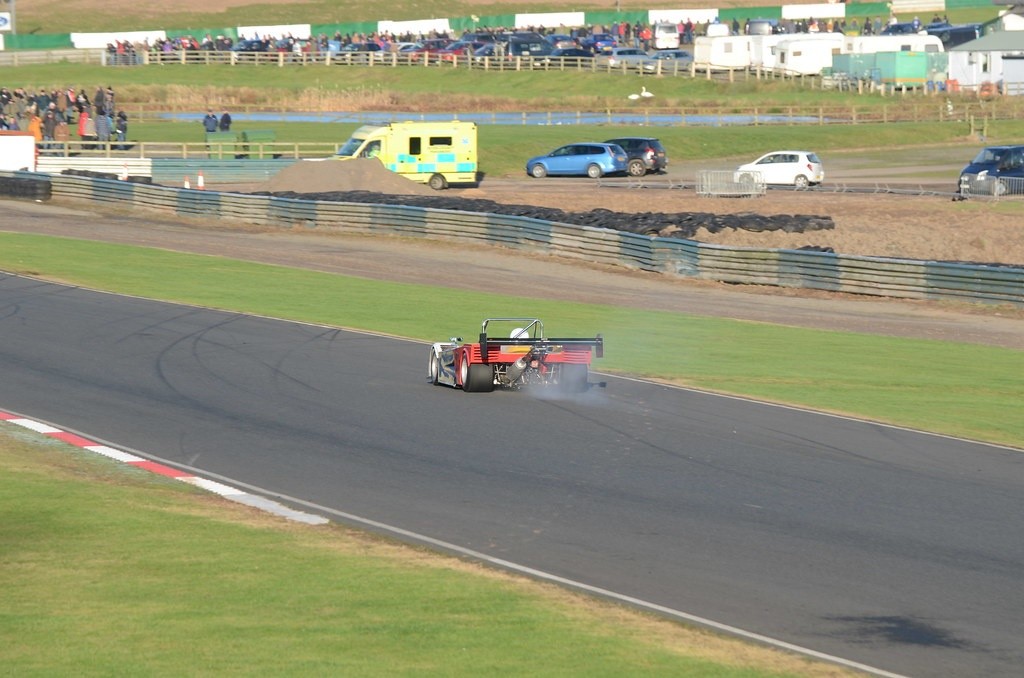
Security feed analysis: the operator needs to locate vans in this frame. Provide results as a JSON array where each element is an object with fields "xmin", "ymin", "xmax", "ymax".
[
  {"xmin": 652, "ymin": 23, "xmax": 680, "ymax": 49},
  {"xmin": 706, "ymin": 20, "xmax": 983, "ymax": 50}
]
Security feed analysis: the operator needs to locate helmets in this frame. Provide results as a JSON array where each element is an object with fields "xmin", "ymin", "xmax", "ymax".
[{"xmin": 509, "ymin": 328, "xmax": 530, "ymax": 338}]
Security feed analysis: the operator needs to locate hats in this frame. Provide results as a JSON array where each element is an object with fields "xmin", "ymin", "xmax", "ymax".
[{"xmin": 208, "ymin": 109, "xmax": 212, "ymax": 112}]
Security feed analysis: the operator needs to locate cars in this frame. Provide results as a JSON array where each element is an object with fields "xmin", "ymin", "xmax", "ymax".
[
  {"xmin": 228, "ymin": 30, "xmax": 694, "ymax": 70},
  {"xmin": 958, "ymin": 145, "xmax": 1024, "ymax": 196}
]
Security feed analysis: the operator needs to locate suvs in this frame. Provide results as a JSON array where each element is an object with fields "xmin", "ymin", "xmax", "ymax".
[
  {"xmin": 733, "ymin": 151, "xmax": 824, "ymax": 188},
  {"xmin": 526, "ymin": 143, "xmax": 628, "ymax": 178},
  {"xmin": 603, "ymin": 137, "xmax": 669, "ymax": 177}
]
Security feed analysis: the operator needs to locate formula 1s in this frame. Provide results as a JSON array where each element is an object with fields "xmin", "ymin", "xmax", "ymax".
[{"xmin": 427, "ymin": 317, "xmax": 607, "ymax": 392}]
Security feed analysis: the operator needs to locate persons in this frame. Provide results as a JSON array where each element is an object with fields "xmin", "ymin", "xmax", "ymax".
[
  {"xmin": 509, "ymin": 328, "xmax": 529, "ymax": 339},
  {"xmin": 203, "ymin": 110, "xmax": 218, "ymax": 151},
  {"xmin": 0, "ymin": 86, "xmax": 128, "ymax": 150},
  {"xmin": 107, "ymin": 30, "xmax": 364, "ymax": 66},
  {"xmin": 219, "ymin": 108, "xmax": 232, "ymax": 133},
  {"xmin": 368, "ymin": 144, "xmax": 380, "ymax": 157},
  {"xmin": 368, "ymin": 12, "xmax": 949, "ymax": 66}
]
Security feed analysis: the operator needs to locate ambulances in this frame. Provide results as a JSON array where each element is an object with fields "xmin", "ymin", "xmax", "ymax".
[{"xmin": 326, "ymin": 121, "xmax": 478, "ymax": 190}]
